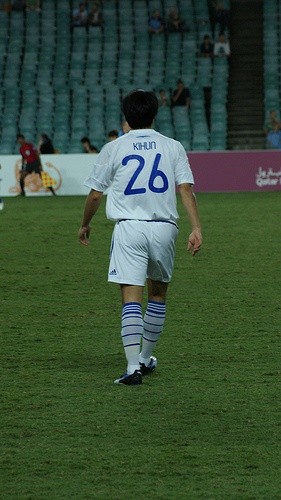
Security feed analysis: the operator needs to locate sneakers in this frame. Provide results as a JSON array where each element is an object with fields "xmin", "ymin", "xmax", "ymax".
[
  {"xmin": 139, "ymin": 356, "xmax": 157, "ymax": 376},
  {"xmin": 114, "ymin": 370, "xmax": 143, "ymax": 385}
]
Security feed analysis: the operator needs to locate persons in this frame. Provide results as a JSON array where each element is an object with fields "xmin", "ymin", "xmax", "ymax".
[
  {"xmin": 108, "ymin": 130, "xmax": 118, "ymax": 142},
  {"xmin": 214, "ymin": 1, "xmax": 236, "ymax": 36},
  {"xmin": 10, "ymin": 0, "xmax": 27, "ymax": 17},
  {"xmin": 156, "ymin": 88, "xmax": 171, "ymax": 112},
  {"xmin": 213, "ymin": 32, "xmax": 231, "ymax": 57},
  {"xmin": 118, "ymin": 121, "xmax": 131, "ymax": 137},
  {"xmin": 171, "ymin": 78, "xmax": 191, "ymax": 109},
  {"xmin": 80, "ymin": 136, "xmax": 99, "ymax": 153},
  {"xmin": 70, "ymin": 3, "xmax": 89, "ymax": 35},
  {"xmin": 199, "ymin": 35, "xmax": 215, "ymax": 61},
  {"xmin": 264, "ymin": 110, "xmax": 281, "ymax": 135},
  {"xmin": 37, "ymin": 132, "xmax": 61, "ymax": 154},
  {"xmin": 87, "ymin": 2, "xmax": 105, "ymax": 34},
  {"xmin": 0, "ymin": 198, "xmax": 4, "ymax": 210},
  {"xmin": 146, "ymin": 8, "xmax": 168, "ymax": 44},
  {"xmin": 15, "ymin": 134, "xmax": 57, "ymax": 197},
  {"xmin": 167, "ymin": 8, "xmax": 190, "ymax": 41},
  {"xmin": 79, "ymin": 90, "xmax": 204, "ymax": 385},
  {"xmin": 266, "ymin": 123, "xmax": 281, "ymax": 149},
  {"xmin": 25, "ymin": 0, "xmax": 43, "ymax": 13}
]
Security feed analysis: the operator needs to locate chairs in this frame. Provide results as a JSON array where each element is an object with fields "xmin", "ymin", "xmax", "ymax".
[{"xmin": 0, "ymin": 0, "xmax": 281, "ymax": 156}]
